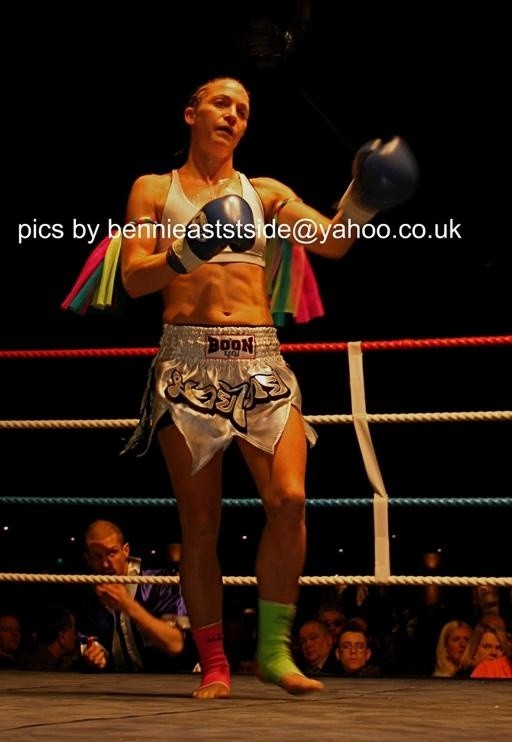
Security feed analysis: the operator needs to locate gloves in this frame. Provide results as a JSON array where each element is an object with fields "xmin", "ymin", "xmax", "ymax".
[
  {"xmin": 165, "ymin": 196, "xmax": 258, "ymax": 282},
  {"xmin": 345, "ymin": 131, "xmax": 423, "ymax": 217}
]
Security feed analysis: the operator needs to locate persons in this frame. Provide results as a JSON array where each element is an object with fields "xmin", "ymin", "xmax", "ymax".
[
  {"xmin": 117, "ymin": 74, "xmax": 431, "ymax": 701},
  {"xmin": 2, "ymin": 517, "xmax": 510, "ymax": 682}
]
